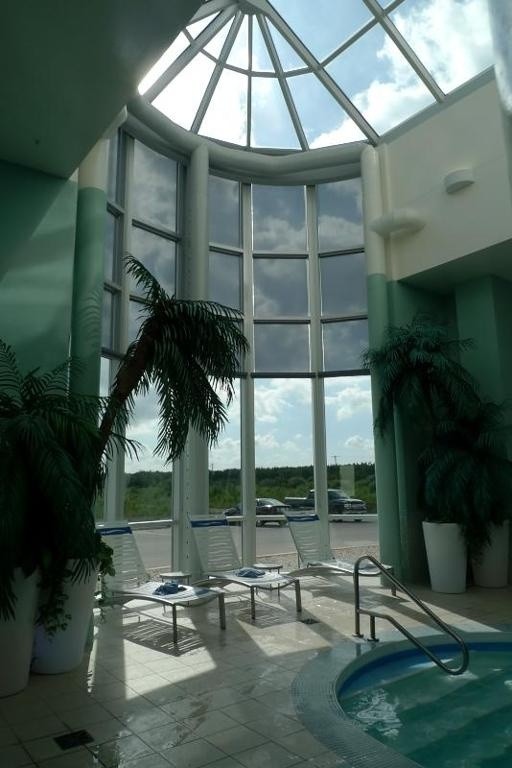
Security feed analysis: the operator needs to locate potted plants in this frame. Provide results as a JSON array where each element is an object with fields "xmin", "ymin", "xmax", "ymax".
[
  {"xmin": 360, "ymin": 308, "xmax": 512, "ymax": 597},
  {"xmin": 0, "ymin": 339, "xmax": 143, "ymax": 698},
  {"xmin": 33, "ymin": 252, "xmax": 251, "ymax": 676}
]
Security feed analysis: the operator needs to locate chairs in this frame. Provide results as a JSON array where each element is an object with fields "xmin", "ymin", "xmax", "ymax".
[
  {"xmin": 186, "ymin": 508, "xmax": 302, "ymax": 620},
  {"xmin": 95, "ymin": 517, "xmax": 228, "ymax": 644},
  {"xmin": 285, "ymin": 512, "xmax": 397, "ymax": 597}
]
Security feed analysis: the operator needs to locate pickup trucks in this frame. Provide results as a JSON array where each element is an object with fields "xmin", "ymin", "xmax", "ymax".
[{"xmin": 284, "ymin": 489, "xmax": 368, "ymax": 522}]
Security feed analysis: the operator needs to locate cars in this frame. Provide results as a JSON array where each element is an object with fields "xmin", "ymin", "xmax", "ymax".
[{"xmin": 224, "ymin": 498, "xmax": 291, "ymax": 527}]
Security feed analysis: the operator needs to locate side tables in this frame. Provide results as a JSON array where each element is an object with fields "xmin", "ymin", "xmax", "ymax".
[
  {"xmin": 159, "ymin": 570, "xmax": 192, "ymax": 607},
  {"xmin": 252, "ymin": 562, "xmax": 284, "ymax": 597}
]
